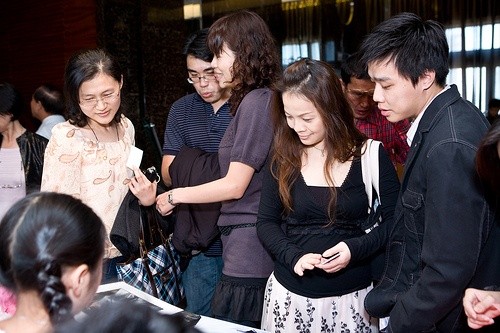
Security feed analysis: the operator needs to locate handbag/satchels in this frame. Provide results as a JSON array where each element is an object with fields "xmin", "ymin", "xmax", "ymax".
[{"xmin": 116, "ymin": 203, "xmax": 185, "ymax": 304}]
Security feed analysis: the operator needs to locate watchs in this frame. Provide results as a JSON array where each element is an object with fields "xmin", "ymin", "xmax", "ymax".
[{"xmin": 168, "ymin": 188, "xmax": 178, "ymax": 205}]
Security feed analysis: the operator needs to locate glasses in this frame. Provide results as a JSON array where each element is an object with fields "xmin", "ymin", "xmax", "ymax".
[
  {"xmin": 345, "ymin": 86, "xmax": 375, "ymax": 100},
  {"xmin": 79, "ymin": 90, "xmax": 122, "ymax": 105},
  {"xmin": 187, "ymin": 74, "xmax": 213, "ymax": 82}
]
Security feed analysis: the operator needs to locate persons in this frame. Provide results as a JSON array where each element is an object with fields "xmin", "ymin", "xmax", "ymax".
[{"xmin": 0, "ymin": 10, "xmax": 500, "ymax": 333}]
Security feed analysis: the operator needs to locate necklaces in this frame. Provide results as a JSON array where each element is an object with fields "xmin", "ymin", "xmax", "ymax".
[
  {"xmin": 312, "ymin": 145, "xmax": 327, "ymax": 156},
  {"xmin": 87, "ymin": 122, "xmax": 119, "ymax": 140}
]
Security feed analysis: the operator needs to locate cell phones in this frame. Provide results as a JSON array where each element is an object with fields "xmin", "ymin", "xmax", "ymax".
[{"xmin": 142, "ymin": 166, "xmax": 156, "ymax": 183}]
